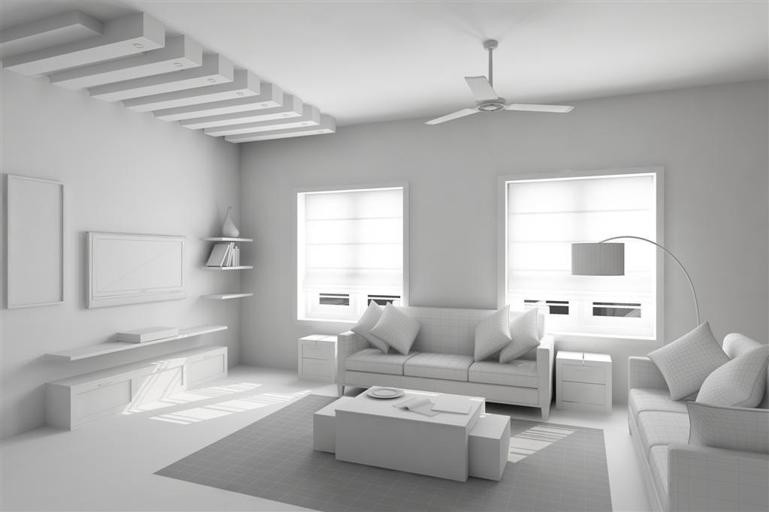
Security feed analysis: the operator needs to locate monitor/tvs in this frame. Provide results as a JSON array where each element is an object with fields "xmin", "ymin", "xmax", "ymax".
[{"xmin": 82, "ymin": 231, "xmax": 188, "ymax": 309}]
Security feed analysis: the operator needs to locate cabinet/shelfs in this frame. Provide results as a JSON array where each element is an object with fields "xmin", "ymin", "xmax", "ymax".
[
  {"xmin": 43, "ymin": 326, "xmax": 226, "ymax": 428},
  {"xmin": 202, "ymin": 237, "xmax": 255, "ymax": 299}
]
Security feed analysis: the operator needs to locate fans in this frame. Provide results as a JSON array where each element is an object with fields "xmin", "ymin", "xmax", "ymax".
[{"xmin": 422, "ymin": 38, "xmax": 575, "ymax": 127}]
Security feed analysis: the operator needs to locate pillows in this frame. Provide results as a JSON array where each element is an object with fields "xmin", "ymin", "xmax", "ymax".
[
  {"xmin": 647, "ymin": 322, "xmax": 731, "ymax": 400},
  {"xmin": 352, "ymin": 298, "xmax": 393, "ymax": 354},
  {"xmin": 697, "ymin": 343, "xmax": 767, "ymax": 408},
  {"xmin": 499, "ymin": 305, "xmax": 546, "ymax": 362},
  {"xmin": 683, "ymin": 399, "xmax": 768, "ymax": 457},
  {"xmin": 369, "ymin": 301, "xmax": 420, "ymax": 354},
  {"xmin": 474, "ymin": 304, "xmax": 511, "ymax": 361}
]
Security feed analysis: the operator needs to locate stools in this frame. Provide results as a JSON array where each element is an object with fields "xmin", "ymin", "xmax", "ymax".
[
  {"xmin": 313, "ymin": 394, "xmax": 354, "ymax": 454},
  {"xmin": 467, "ymin": 413, "xmax": 511, "ymax": 480}
]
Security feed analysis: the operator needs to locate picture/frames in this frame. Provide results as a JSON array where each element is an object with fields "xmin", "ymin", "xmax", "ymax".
[{"xmin": 3, "ymin": 172, "xmax": 66, "ymax": 310}]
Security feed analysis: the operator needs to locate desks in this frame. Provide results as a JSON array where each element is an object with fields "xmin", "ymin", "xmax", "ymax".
[
  {"xmin": 554, "ymin": 351, "xmax": 613, "ymax": 411},
  {"xmin": 297, "ymin": 333, "xmax": 337, "ymax": 382}
]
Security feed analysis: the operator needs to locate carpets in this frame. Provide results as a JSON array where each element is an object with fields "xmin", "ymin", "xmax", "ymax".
[{"xmin": 156, "ymin": 393, "xmax": 611, "ymax": 512}]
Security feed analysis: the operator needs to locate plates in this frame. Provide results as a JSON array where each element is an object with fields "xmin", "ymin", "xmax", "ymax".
[{"xmin": 367, "ymin": 388, "xmax": 405, "ymax": 398}]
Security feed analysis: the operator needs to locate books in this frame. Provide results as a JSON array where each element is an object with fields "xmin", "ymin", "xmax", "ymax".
[
  {"xmin": 205, "ymin": 242, "xmax": 241, "ymax": 268},
  {"xmin": 432, "ymin": 393, "xmax": 473, "ymax": 416},
  {"xmin": 393, "ymin": 393, "xmax": 443, "ymax": 418}
]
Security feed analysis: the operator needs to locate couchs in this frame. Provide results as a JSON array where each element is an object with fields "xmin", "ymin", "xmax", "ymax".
[
  {"xmin": 337, "ymin": 307, "xmax": 553, "ymax": 422},
  {"xmin": 628, "ymin": 331, "xmax": 769, "ymax": 512}
]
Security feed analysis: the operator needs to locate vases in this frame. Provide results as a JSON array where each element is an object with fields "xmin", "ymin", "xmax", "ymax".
[{"xmin": 222, "ymin": 206, "xmax": 239, "ymax": 237}]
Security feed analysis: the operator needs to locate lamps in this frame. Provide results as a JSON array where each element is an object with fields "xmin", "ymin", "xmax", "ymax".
[{"xmin": 571, "ymin": 235, "xmax": 702, "ymax": 327}]
